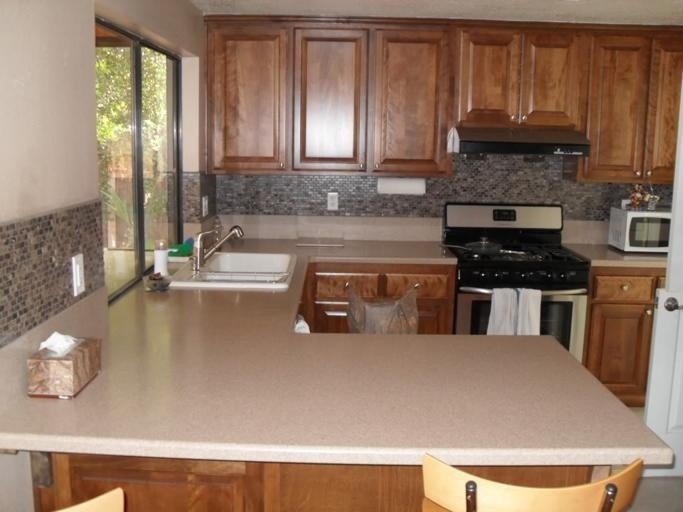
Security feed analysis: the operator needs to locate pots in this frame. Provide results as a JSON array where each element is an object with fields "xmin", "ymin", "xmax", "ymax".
[{"xmin": 438, "ymin": 239, "xmax": 498, "ymax": 261}]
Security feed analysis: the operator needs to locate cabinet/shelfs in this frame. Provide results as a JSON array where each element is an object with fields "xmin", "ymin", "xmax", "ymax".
[
  {"xmin": 212, "ymin": 215, "xmax": 222, "ymax": 244},
  {"xmin": 154, "ymin": 239, "xmax": 168, "ymax": 277}
]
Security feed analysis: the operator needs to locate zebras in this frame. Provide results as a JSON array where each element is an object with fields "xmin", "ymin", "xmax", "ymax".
[{"xmin": 458, "ymin": 283, "xmax": 587, "ymax": 367}]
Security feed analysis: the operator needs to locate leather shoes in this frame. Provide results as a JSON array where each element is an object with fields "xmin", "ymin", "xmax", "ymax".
[{"xmin": 167, "ymin": 237, "xmax": 193, "ymax": 256}]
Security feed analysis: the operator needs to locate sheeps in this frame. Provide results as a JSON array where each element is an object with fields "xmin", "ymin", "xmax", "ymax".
[{"xmin": 192, "ymin": 225, "xmax": 245, "ymax": 266}]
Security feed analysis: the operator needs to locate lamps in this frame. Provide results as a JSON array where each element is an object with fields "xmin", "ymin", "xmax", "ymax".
[{"xmin": 422, "ymin": 450, "xmax": 644, "ymax": 512}]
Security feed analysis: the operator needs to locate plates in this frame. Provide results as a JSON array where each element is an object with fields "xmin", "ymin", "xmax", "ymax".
[
  {"xmin": 582, "ymin": 267, "xmax": 666, "ymax": 407},
  {"xmin": 204, "ymin": 16, "xmax": 286, "ymax": 175},
  {"xmin": 454, "ymin": 20, "xmax": 592, "ymax": 144},
  {"xmin": 285, "ymin": 18, "xmax": 454, "ymax": 178},
  {"xmin": 563, "ymin": 25, "xmax": 683, "ymax": 185},
  {"xmin": 312, "ymin": 263, "xmax": 456, "ymax": 335}
]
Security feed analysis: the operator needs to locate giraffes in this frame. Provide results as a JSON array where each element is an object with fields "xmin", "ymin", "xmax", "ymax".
[{"xmin": 444, "ymin": 242, "xmax": 590, "ymax": 288}]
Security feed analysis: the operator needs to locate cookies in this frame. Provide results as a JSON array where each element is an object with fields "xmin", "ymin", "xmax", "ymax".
[{"xmin": 608, "ymin": 205, "xmax": 671, "ymax": 253}]
